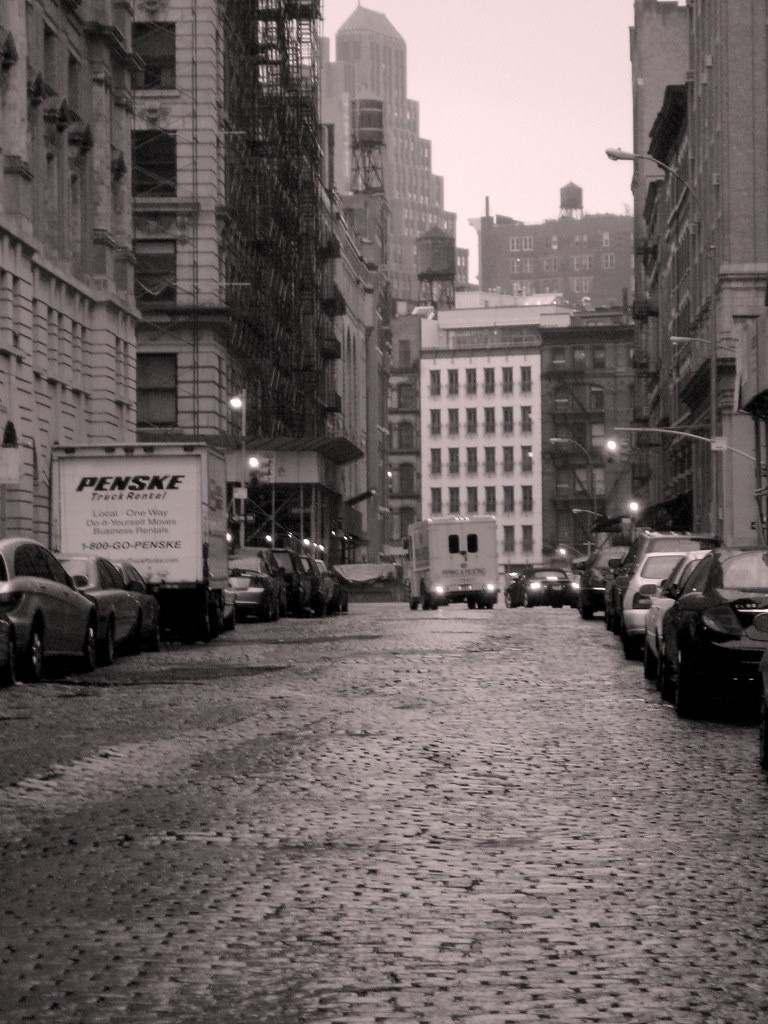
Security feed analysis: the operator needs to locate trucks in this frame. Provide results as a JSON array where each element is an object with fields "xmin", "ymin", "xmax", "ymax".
[{"xmin": 46, "ymin": 441, "xmax": 205, "ymax": 638}]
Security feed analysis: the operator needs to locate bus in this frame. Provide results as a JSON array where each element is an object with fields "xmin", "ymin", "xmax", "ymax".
[{"xmin": 401, "ymin": 514, "xmax": 500, "ymax": 611}]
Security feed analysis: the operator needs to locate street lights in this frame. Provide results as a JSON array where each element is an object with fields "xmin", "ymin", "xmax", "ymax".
[
  {"xmin": 230, "ymin": 387, "xmax": 247, "ymax": 548},
  {"xmin": 549, "ymin": 437, "xmax": 599, "ymax": 510},
  {"xmin": 605, "ymin": 147, "xmax": 719, "ymax": 535}
]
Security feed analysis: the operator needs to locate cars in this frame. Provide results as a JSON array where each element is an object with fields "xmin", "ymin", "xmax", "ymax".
[
  {"xmin": 0, "ymin": 537, "xmax": 99, "ymax": 684},
  {"xmin": 223, "ymin": 545, "xmax": 350, "ymax": 629},
  {"xmin": 51, "ymin": 551, "xmax": 142, "ymax": 664},
  {"xmin": 111, "ymin": 562, "xmax": 164, "ymax": 653},
  {"xmin": 505, "ymin": 525, "xmax": 768, "ymax": 719}
]
